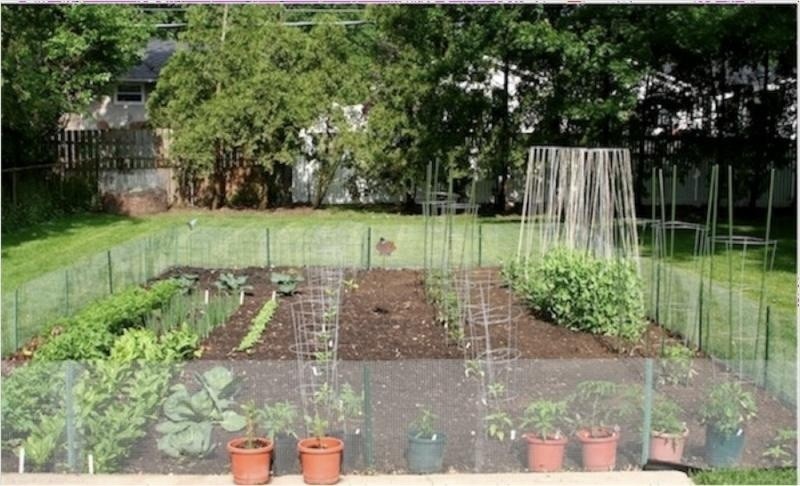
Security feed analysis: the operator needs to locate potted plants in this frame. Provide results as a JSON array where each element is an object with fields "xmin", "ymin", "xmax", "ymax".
[
  {"xmin": 403, "ymin": 405, "xmax": 446, "ymax": 473},
  {"xmin": 564, "ymin": 379, "xmax": 632, "ymax": 472},
  {"xmin": 631, "ymin": 385, "xmax": 691, "ymax": 464},
  {"xmin": 296, "ymin": 410, "xmax": 345, "ymax": 486},
  {"xmin": 517, "ymin": 399, "xmax": 569, "ymax": 473},
  {"xmin": 695, "ymin": 379, "xmax": 759, "ymax": 469},
  {"xmin": 226, "ymin": 399, "xmax": 274, "ymax": 485}
]
